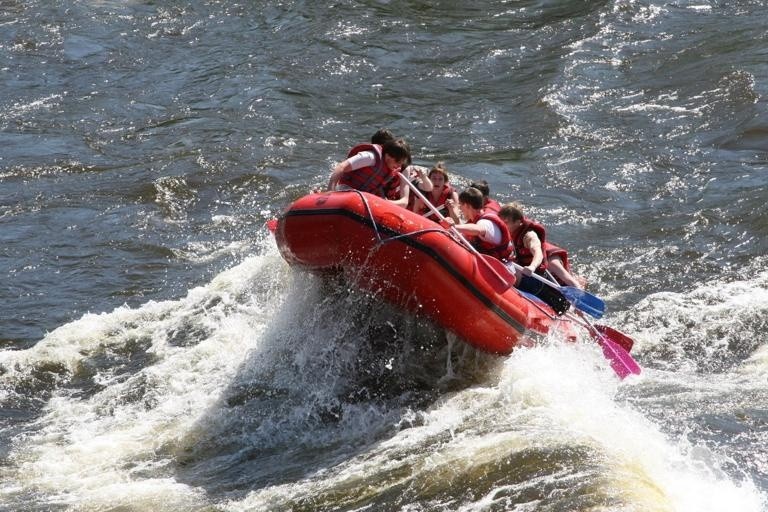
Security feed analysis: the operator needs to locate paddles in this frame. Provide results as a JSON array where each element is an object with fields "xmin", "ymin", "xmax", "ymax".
[
  {"xmin": 546, "ymin": 269, "xmax": 641, "ymax": 380},
  {"xmin": 512, "ymin": 262, "xmax": 604, "ymax": 319},
  {"xmin": 399, "ymin": 172, "xmax": 516, "ymax": 294}
]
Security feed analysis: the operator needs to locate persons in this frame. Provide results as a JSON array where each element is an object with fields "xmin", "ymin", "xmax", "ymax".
[{"xmin": 327, "ymin": 129, "xmax": 582, "ymax": 290}]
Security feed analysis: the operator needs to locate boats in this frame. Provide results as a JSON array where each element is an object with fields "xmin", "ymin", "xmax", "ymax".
[{"xmin": 272, "ymin": 189, "xmax": 577, "ymax": 361}]
